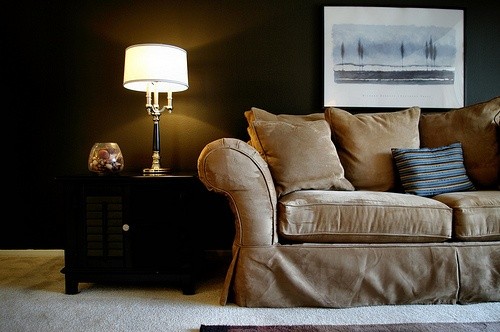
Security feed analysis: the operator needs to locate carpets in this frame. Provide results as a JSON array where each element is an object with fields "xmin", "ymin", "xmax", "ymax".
[{"xmin": 199, "ymin": 322, "xmax": 499, "ymax": 332}]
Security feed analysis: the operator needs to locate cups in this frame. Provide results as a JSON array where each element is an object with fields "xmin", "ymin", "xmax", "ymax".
[{"xmin": 88, "ymin": 141, "xmax": 124, "ymax": 177}]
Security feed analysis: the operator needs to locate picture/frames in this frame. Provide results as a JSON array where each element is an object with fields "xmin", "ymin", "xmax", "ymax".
[{"xmin": 320, "ymin": 3, "xmax": 467, "ymax": 111}]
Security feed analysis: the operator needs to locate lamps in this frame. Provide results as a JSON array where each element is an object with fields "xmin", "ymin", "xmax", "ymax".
[{"xmin": 124, "ymin": 43, "xmax": 190, "ymax": 172}]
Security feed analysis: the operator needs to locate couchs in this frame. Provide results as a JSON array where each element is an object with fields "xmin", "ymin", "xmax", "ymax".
[{"xmin": 198, "ymin": 97, "xmax": 500, "ymax": 308}]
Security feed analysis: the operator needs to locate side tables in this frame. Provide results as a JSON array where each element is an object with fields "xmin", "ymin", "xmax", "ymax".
[{"xmin": 61, "ymin": 170, "xmax": 226, "ymax": 296}]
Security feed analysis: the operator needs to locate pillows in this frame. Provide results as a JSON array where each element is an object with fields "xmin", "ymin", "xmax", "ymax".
[
  {"xmin": 325, "ymin": 105, "xmax": 428, "ymax": 192},
  {"xmin": 247, "ymin": 107, "xmax": 357, "ymax": 198},
  {"xmin": 419, "ymin": 97, "xmax": 500, "ymax": 190},
  {"xmin": 389, "ymin": 143, "xmax": 477, "ymax": 198}
]
